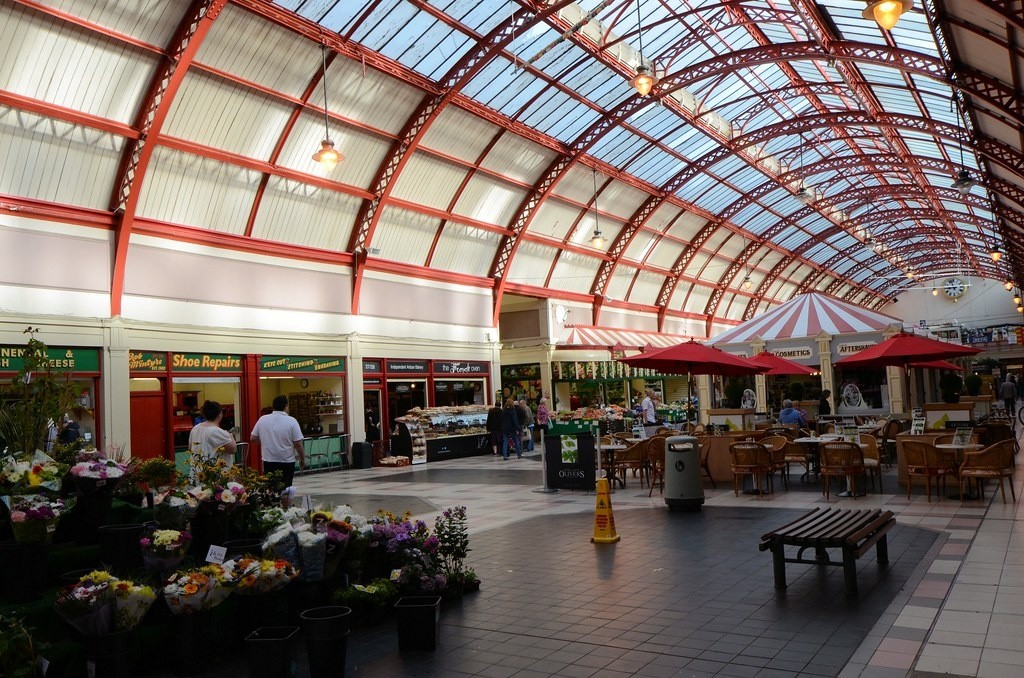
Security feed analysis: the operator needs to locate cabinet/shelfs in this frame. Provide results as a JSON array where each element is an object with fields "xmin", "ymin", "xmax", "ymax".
[{"xmin": 309, "ymin": 396, "xmax": 342, "ymax": 415}]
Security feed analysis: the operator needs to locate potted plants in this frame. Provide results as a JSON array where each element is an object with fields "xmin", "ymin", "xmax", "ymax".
[
  {"xmin": 965, "ymin": 374, "xmax": 983, "ymax": 397},
  {"xmin": 939, "ymin": 373, "xmax": 963, "ymax": 404},
  {"xmin": 333, "ymin": 577, "xmax": 396, "ymax": 626},
  {"xmin": 460, "ymin": 566, "xmax": 481, "ymax": 593}
]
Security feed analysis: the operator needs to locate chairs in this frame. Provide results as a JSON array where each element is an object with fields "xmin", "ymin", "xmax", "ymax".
[
  {"xmin": 294, "ymin": 433, "xmax": 352, "ymax": 477},
  {"xmin": 593, "ymin": 416, "xmax": 1020, "ymax": 505}
]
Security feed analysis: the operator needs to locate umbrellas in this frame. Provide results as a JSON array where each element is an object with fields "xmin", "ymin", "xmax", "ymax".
[
  {"xmin": 744, "ymin": 348, "xmax": 822, "ymax": 418},
  {"xmin": 835, "ymin": 329, "xmax": 986, "ymax": 412},
  {"xmin": 616, "ymin": 337, "xmax": 773, "ymax": 438}
]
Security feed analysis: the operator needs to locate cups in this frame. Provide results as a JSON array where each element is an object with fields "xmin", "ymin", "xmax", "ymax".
[
  {"xmin": 746, "ymin": 438, "xmax": 752, "ymax": 445},
  {"xmin": 811, "ymin": 431, "xmax": 815, "ymax": 440}
]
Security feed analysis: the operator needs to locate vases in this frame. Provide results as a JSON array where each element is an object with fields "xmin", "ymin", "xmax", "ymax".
[
  {"xmin": 97, "ymin": 524, "xmax": 145, "ymax": 571},
  {"xmin": 393, "ymin": 596, "xmax": 443, "ymax": 655},
  {"xmin": 244, "ymin": 606, "xmax": 352, "ymax": 678},
  {"xmin": 221, "ymin": 540, "xmax": 264, "ymax": 555},
  {"xmin": 60, "ymin": 568, "xmax": 101, "ymax": 583},
  {"xmin": 442, "ymin": 583, "xmax": 464, "ymax": 601},
  {"xmin": 80, "ymin": 630, "xmax": 140, "ymax": 678}
]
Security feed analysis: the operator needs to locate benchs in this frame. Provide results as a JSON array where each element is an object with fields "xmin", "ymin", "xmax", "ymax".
[{"xmin": 759, "ymin": 506, "xmax": 896, "ymax": 600}]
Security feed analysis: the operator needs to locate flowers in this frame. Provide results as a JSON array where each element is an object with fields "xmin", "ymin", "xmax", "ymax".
[{"xmin": 0, "ymin": 445, "xmax": 481, "ymax": 637}]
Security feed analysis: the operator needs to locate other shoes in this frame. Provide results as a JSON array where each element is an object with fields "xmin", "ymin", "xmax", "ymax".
[
  {"xmin": 493, "ymin": 453, "xmax": 497, "ymax": 457},
  {"xmin": 503, "ymin": 458, "xmax": 509, "ymax": 460},
  {"xmin": 518, "ymin": 456, "xmax": 521, "ymax": 459}
]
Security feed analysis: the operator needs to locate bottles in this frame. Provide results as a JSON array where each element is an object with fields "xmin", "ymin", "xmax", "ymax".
[{"xmin": 669, "ymin": 408, "xmax": 687, "ymax": 421}]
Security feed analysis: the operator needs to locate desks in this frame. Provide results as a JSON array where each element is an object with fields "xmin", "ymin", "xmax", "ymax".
[
  {"xmin": 793, "ymin": 436, "xmax": 836, "ymax": 485},
  {"xmin": 594, "ymin": 445, "xmax": 627, "ymax": 488},
  {"xmin": 934, "ymin": 443, "xmax": 983, "ymax": 500},
  {"xmin": 696, "ymin": 429, "xmax": 766, "ymax": 482},
  {"xmin": 825, "ymin": 444, "xmax": 871, "ymax": 498},
  {"xmin": 735, "ymin": 444, "xmax": 773, "ymax": 494},
  {"xmin": 616, "ymin": 436, "xmax": 649, "ymax": 479},
  {"xmin": 859, "ymin": 425, "xmax": 883, "ymax": 435}
]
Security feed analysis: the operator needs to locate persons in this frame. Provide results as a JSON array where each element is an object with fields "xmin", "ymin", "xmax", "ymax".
[
  {"xmin": 642, "ymin": 390, "xmax": 656, "ymax": 426},
  {"xmin": 778, "ymin": 399, "xmax": 809, "ymax": 437},
  {"xmin": 965, "ymin": 371, "xmax": 982, "ymax": 396},
  {"xmin": 723, "ymin": 379, "xmax": 741, "ymax": 408},
  {"xmin": 537, "ymin": 397, "xmax": 550, "ymax": 444},
  {"xmin": 194, "ymin": 406, "xmax": 207, "ymax": 425},
  {"xmin": 250, "ymin": 394, "xmax": 305, "ymax": 494},
  {"xmin": 188, "ymin": 400, "xmax": 237, "ymax": 484},
  {"xmin": 939, "ymin": 370, "xmax": 963, "ymax": 403},
  {"xmin": 691, "ymin": 392, "xmax": 699, "ymax": 413},
  {"xmin": 365, "ymin": 404, "xmax": 380, "ymax": 441},
  {"xmin": 819, "ymin": 390, "xmax": 831, "ymax": 415},
  {"xmin": 570, "ymin": 392, "xmax": 589, "ymax": 410},
  {"xmin": 58, "ymin": 420, "xmax": 80, "ymax": 447},
  {"xmin": 999, "ymin": 373, "xmax": 1024, "ymax": 416},
  {"xmin": 486, "ymin": 398, "xmax": 535, "ymax": 460}
]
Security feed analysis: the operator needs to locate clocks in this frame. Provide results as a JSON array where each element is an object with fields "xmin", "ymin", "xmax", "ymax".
[{"xmin": 940, "ymin": 277, "xmax": 967, "ymax": 303}]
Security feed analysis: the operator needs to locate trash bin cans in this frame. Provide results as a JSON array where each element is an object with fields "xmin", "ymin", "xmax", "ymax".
[
  {"xmin": 664, "ymin": 436, "xmax": 705, "ymax": 510},
  {"xmin": 351, "ymin": 443, "xmax": 373, "ymax": 468}
]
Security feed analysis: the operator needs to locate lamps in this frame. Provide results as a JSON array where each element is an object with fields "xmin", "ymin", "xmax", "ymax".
[
  {"xmin": 312, "ymin": 43, "xmax": 345, "ymax": 164},
  {"xmin": 1002, "ymin": 244, "xmax": 1024, "ymax": 313},
  {"xmin": 905, "ymin": 237, "xmax": 913, "ymax": 279},
  {"xmin": 989, "ymin": 207, "xmax": 1002, "ymax": 261},
  {"xmin": 628, "ymin": 0, "xmax": 659, "ymax": 97},
  {"xmin": 950, "ymin": 99, "xmax": 976, "ymax": 195},
  {"xmin": 588, "ymin": 169, "xmax": 608, "ymax": 250},
  {"xmin": 742, "ymin": 235, "xmax": 753, "ymax": 290},
  {"xmin": 796, "ymin": 132, "xmax": 813, "ymax": 205},
  {"xmin": 865, "ymin": 189, "xmax": 875, "ymax": 251},
  {"xmin": 932, "ymin": 251, "xmax": 939, "ymax": 296},
  {"xmin": 862, "ymin": 0, "xmax": 915, "ymax": 31}
]
